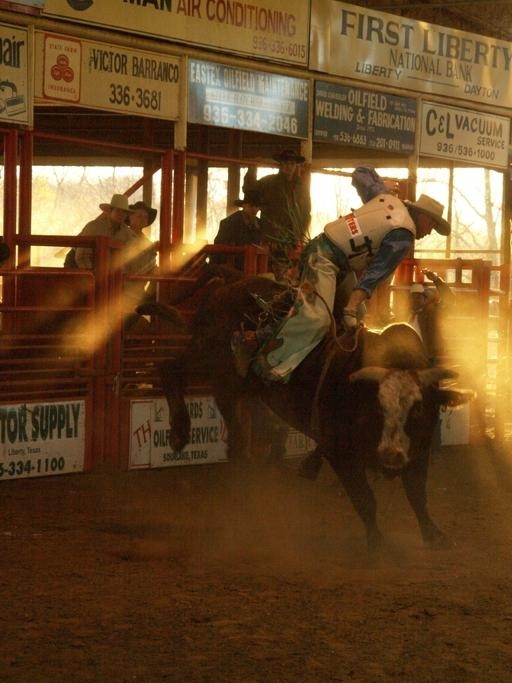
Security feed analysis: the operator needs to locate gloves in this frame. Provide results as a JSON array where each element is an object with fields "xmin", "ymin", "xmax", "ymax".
[{"xmin": 340, "ymin": 307, "xmax": 358, "ymax": 333}]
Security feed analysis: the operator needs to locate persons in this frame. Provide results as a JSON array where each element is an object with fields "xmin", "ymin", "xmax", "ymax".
[
  {"xmin": 213, "ymin": 190, "xmax": 268, "ymax": 244},
  {"xmin": 229, "ymin": 192, "xmax": 451, "ymax": 378},
  {"xmin": 121, "ymin": 200, "xmax": 157, "ymax": 299},
  {"xmin": 243, "ymin": 148, "xmax": 312, "ymax": 238},
  {"xmin": 63, "ymin": 193, "xmax": 138, "ymax": 268},
  {"xmin": 408, "ymin": 268, "xmax": 456, "ymax": 354}
]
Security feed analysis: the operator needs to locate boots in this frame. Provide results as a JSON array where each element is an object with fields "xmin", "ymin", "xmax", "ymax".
[{"xmin": 230, "ymin": 329, "xmax": 265, "ymax": 379}]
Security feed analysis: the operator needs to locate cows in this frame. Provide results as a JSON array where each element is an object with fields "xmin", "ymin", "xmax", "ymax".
[{"xmin": 134, "ymin": 273, "xmax": 478, "ymax": 557}]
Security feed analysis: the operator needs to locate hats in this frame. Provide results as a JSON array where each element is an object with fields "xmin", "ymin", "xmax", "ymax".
[
  {"xmin": 126, "ymin": 200, "xmax": 157, "ymax": 226},
  {"xmin": 99, "ymin": 193, "xmax": 137, "ymax": 216},
  {"xmin": 402, "ymin": 192, "xmax": 451, "ymax": 236},
  {"xmin": 272, "ymin": 147, "xmax": 305, "ymax": 164},
  {"xmin": 233, "ymin": 190, "xmax": 272, "ymax": 207}
]
